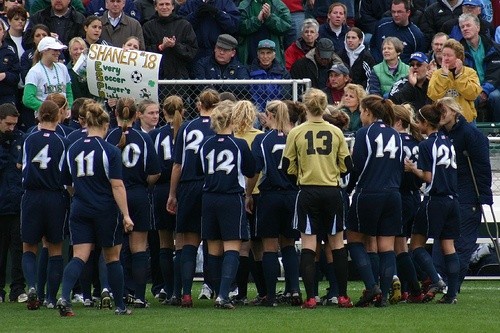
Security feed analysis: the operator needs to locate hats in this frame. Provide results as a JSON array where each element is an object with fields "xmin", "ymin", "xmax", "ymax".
[
  {"xmin": 37, "ymin": 36, "xmax": 67, "ymax": 52},
  {"xmin": 258, "ymin": 39, "xmax": 276, "ymax": 51},
  {"xmin": 217, "ymin": 34, "xmax": 238, "ymax": 50},
  {"xmin": 329, "ymin": 65, "xmax": 349, "ymax": 75},
  {"xmin": 463, "ymin": 0, "xmax": 481, "ymax": 5},
  {"xmin": 318, "ymin": 38, "xmax": 334, "ymax": 59},
  {"xmin": 409, "ymin": 51, "xmax": 429, "ymax": 64}
]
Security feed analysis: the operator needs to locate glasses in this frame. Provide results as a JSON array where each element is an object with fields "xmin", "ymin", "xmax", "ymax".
[
  {"xmin": 410, "ymin": 62, "xmax": 425, "ymax": 67},
  {"xmin": 9, "ymin": 0, "xmax": 24, "ymax": 4}
]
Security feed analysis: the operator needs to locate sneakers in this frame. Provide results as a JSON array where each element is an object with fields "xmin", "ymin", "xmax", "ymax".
[
  {"xmin": 114, "ymin": 306, "xmax": 131, "ymax": 315},
  {"xmin": 0, "ymin": 278, "xmax": 459, "ymax": 309},
  {"xmin": 57, "ymin": 297, "xmax": 75, "ymax": 316}
]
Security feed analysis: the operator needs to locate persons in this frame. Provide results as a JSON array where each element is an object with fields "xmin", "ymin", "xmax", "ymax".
[{"xmin": 0, "ymin": 0, "xmax": 500, "ymax": 315}]
